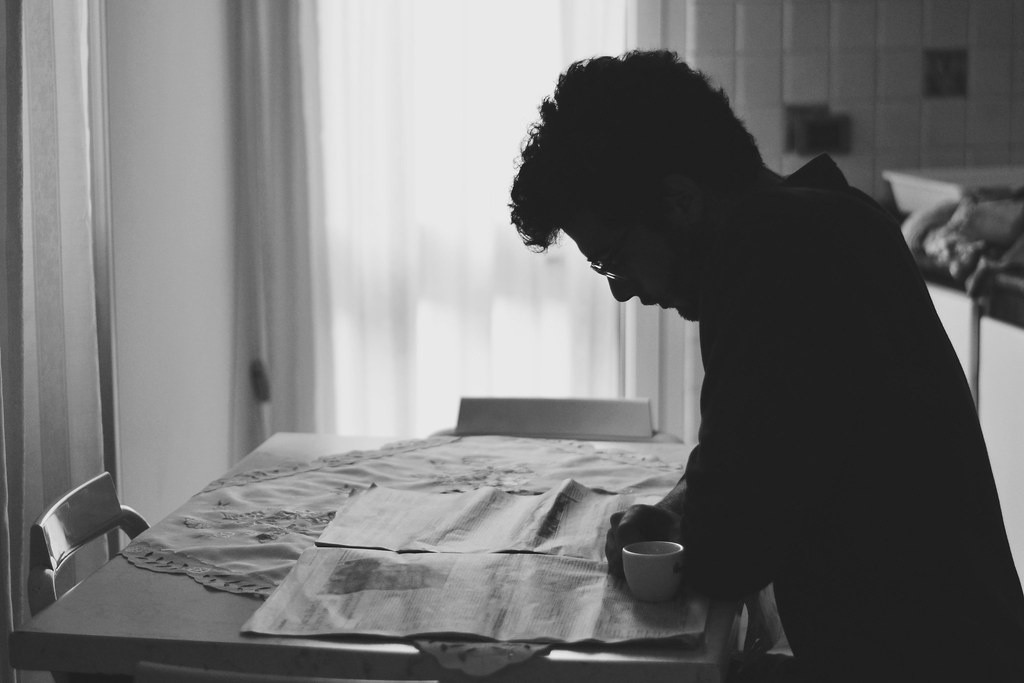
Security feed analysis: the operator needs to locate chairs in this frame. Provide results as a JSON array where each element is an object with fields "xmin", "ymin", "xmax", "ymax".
[
  {"xmin": 25, "ymin": 469, "xmax": 158, "ymax": 682},
  {"xmin": 452, "ymin": 396, "xmax": 660, "ymax": 443}
]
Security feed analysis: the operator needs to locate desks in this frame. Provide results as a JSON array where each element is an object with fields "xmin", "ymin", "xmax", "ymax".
[{"xmin": 9, "ymin": 428, "xmax": 766, "ymax": 682}]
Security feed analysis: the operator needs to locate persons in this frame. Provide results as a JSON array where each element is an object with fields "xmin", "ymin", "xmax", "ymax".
[{"xmin": 505, "ymin": 48, "xmax": 1024, "ymax": 682}]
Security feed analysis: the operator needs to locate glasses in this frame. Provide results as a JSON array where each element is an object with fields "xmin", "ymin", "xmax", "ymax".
[{"xmin": 589, "ymin": 188, "xmax": 666, "ymax": 283}]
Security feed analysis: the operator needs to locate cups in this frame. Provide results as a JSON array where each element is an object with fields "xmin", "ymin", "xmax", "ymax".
[{"xmin": 622, "ymin": 540, "xmax": 684, "ymax": 603}]
